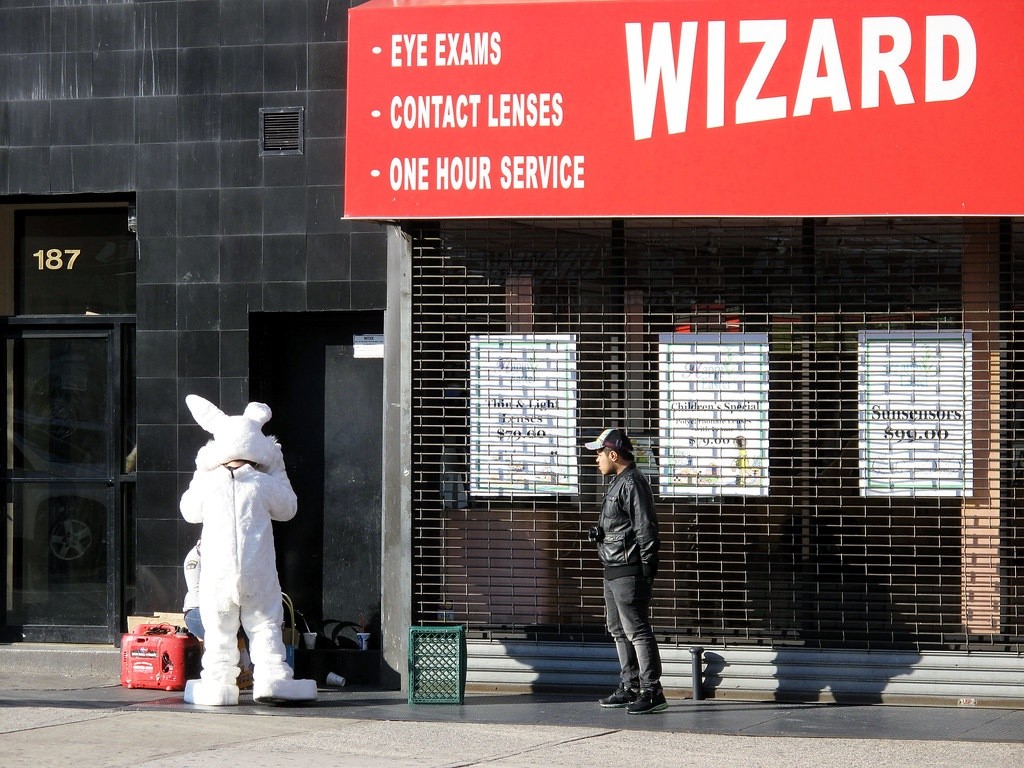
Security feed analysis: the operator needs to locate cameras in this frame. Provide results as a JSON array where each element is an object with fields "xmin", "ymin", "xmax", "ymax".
[{"xmin": 588, "ymin": 526, "xmax": 605, "ymax": 542}]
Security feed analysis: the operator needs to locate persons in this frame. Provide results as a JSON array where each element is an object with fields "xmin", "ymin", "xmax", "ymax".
[
  {"xmin": 585, "ymin": 429, "xmax": 669, "ymax": 714},
  {"xmin": 179, "ymin": 395, "xmax": 318, "ymax": 704}
]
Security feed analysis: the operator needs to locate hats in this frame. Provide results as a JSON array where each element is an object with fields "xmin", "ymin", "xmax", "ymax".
[{"xmin": 585, "ymin": 428, "xmax": 634, "ymax": 452}]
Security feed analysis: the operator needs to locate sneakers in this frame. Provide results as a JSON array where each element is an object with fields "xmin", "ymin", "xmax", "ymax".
[
  {"xmin": 599, "ymin": 682, "xmax": 640, "ymax": 708},
  {"xmin": 625, "ymin": 685, "xmax": 669, "ymax": 715}
]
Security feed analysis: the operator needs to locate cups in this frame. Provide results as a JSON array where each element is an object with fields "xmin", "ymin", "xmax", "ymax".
[
  {"xmin": 326, "ymin": 672, "xmax": 345, "ymax": 686},
  {"xmin": 357, "ymin": 633, "xmax": 370, "ymax": 650},
  {"xmin": 303, "ymin": 633, "xmax": 317, "ymax": 649}
]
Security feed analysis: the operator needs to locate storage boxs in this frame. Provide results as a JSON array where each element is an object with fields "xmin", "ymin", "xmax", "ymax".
[
  {"xmin": 197, "ymin": 636, "xmax": 253, "ymax": 690},
  {"xmin": 407, "ymin": 625, "xmax": 467, "ymax": 705},
  {"xmin": 126, "ymin": 612, "xmax": 187, "ymax": 636}
]
